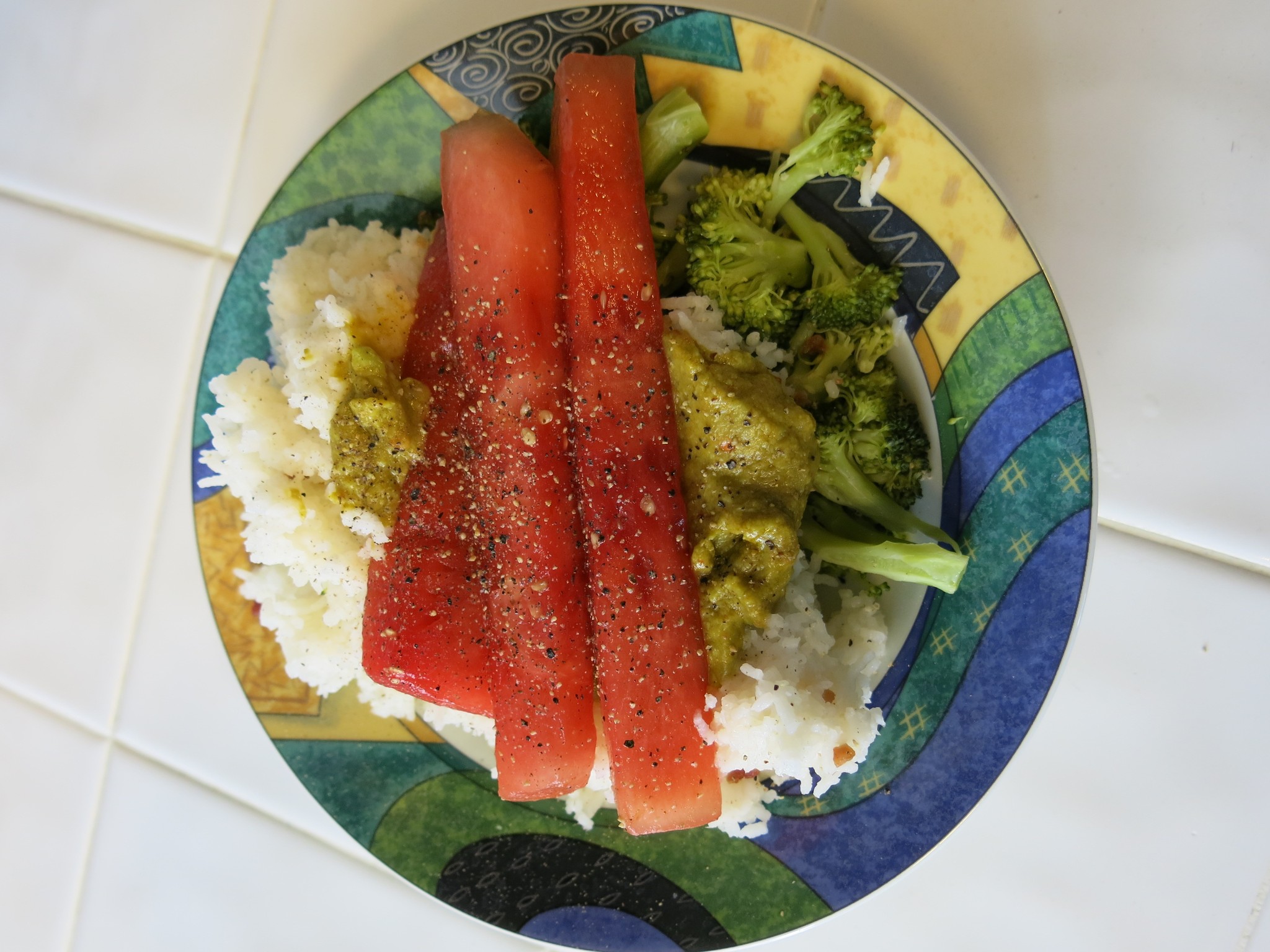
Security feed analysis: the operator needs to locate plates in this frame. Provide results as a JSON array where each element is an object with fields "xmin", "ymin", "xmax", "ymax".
[{"xmin": 193, "ymin": 1, "xmax": 1099, "ymax": 952}]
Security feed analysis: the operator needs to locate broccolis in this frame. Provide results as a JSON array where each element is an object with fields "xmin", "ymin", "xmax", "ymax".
[{"xmin": 634, "ymin": 80, "xmax": 971, "ymax": 598}]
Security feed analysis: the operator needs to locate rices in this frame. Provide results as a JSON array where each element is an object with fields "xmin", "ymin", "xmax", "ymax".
[{"xmin": 194, "ymin": 210, "xmax": 885, "ymax": 839}]
